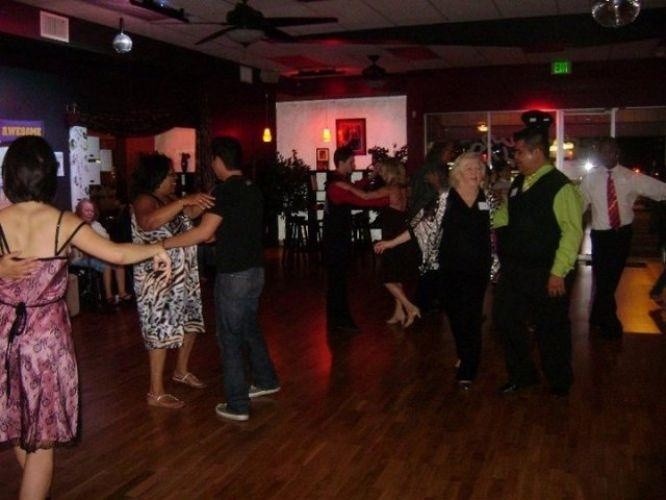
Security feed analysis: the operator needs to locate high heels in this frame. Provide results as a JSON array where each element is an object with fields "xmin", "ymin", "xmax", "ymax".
[
  {"xmin": 404, "ymin": 305, "xmax": 422, "ymax": 328},
  {"xmin": 386, "ymin": 312, "xmax": 405, "ymax": 325}
]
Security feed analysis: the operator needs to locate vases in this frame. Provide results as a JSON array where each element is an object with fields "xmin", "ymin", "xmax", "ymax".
[{"xmin": 180, "ymin": 154, "xmax": 188, "ymax": 172}]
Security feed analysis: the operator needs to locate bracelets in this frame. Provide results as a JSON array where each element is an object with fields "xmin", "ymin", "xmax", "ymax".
[{"xmin": 162, "ymin": 237, "xmax": 166, "ymax": 247}]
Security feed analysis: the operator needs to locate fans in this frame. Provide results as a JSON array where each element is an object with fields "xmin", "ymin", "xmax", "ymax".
[{"xmin": 152, "ymin": 0, "xmax": 338, "ymax": 46}]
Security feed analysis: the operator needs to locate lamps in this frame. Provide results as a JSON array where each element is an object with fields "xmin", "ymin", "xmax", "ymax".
[
  {"xmin": 591, "ymin": 1, "xmax": 640, "ymax": 30},
  {"xmin": 226, "ymin": 27, "xmax": 264, "ymax": 45},
  {"xmin": 361, "ymin": 54, "xmax": 391, "ymax": 88},
  {"xmin": 112, "ymin": 17, "xmax": 134, "ymax": 53}
]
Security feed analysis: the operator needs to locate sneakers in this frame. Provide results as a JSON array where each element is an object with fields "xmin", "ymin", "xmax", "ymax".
[
  {"xmin": 248, "ymin": 384, "xmax": 281, "ymax": 398},
  {"xmin": 215, "ymin": 402, "xmax": 250, "ymax": 421}
]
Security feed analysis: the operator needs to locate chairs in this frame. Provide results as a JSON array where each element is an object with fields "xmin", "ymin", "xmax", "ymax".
[{"xmin": 280, "ymin": 189, "xmax": 372, "ymax": 280}]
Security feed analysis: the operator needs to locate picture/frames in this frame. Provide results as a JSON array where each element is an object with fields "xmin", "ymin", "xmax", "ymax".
[
  {"xmin": 315, "ymin": 148, "xmax": 329, "ymax": 170},
  {"xmin": 335, "ymin": 118, "xmax": 367, "ymax": 156}
]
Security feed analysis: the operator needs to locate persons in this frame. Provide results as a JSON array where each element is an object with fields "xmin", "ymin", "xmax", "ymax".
[
  {"xmin": 0, "ymin": 136, "xmax": 171, "ymax": 500},
  {"xmin": 157, "ymin": 135, "xmax": 282, "ymax": 422},
  {"xmin": 71, "ymin": 199, "xmax": 132, "ymax": 307},
  {"xmin": 128, "ymin": 154, "xmax": 217, "ymax": 411},
  {"xmin": 322, "ymin": 128, "xmax": 666, "ymax": 399},
  {"xmin": 0, "ymin": 252, "xmax": 41, "ymax": 279}
]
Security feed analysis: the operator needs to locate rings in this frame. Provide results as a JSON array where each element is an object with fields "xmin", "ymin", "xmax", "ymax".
[{"xmin": 199, "ymin": 199, "xmax": 202, "ymax": 203}]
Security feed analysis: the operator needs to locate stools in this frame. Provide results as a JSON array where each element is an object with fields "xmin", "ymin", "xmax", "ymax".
[{"xmin": 72, "ymin": 265, "xmax": 105, "ymax": 310}]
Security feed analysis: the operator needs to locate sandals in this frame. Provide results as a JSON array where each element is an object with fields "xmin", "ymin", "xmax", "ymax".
[
  {"xmin": 146, "ymin": 392, "xmax": 184, "ymax": 410},
  {"xmin": 172, "ymin": 369, "xmax": 207, "ymax": 389},
  {"xmin": 119, "ymin": 294, "xmax": 135, "ymax": 303},
  {"xmin": 106, "ymin": 296, "xmax": 123, "ymax": 306}
]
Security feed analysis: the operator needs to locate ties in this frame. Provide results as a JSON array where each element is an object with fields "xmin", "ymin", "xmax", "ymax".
[{"xmin": 606, "ymin": 171, "xmax": 620, "ymax": 233}]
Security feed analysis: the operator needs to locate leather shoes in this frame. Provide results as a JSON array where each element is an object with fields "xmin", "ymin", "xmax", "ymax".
[
  {"xmin": 497, "ymin": 376, "xmax": 541, "ymax": 395},
  {"xmin": 459, "ymin": 380, "xmax": 472, "ymax": 391},
  {"xmin": 338, "ymin": 324, "xmax": 359, "ymax": 333}
]
